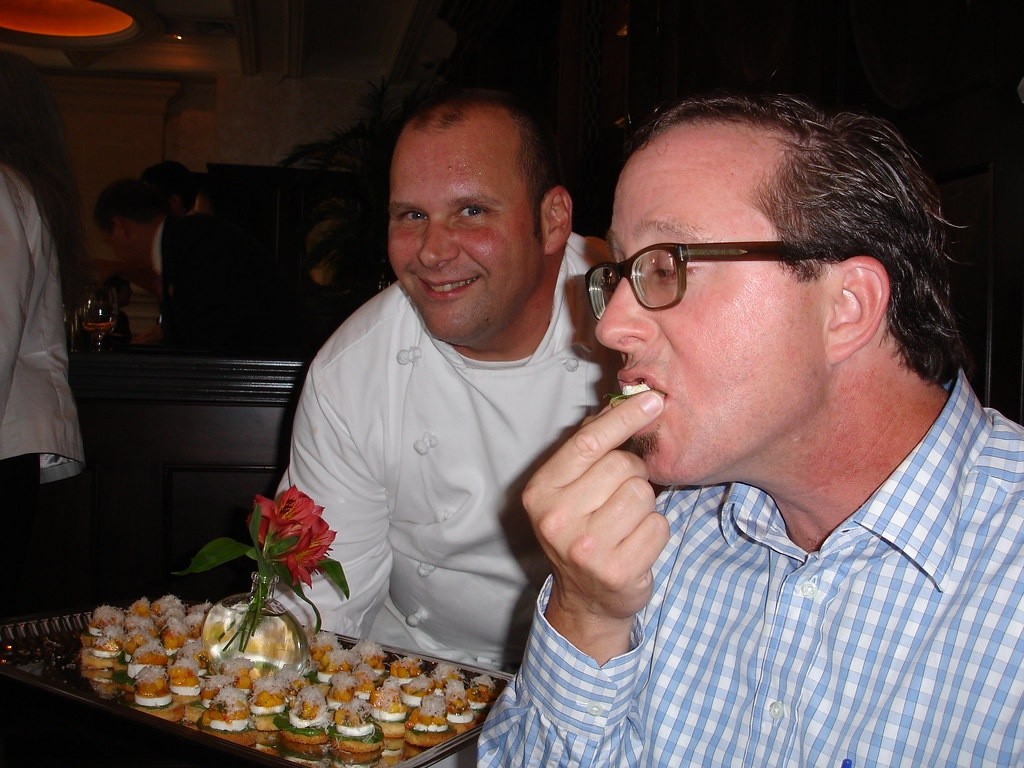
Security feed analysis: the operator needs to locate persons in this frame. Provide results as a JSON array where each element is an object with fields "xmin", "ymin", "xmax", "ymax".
[
  {"xmin": 81, "ymin": 158, "xmax": 398, "ymax": 357},
  {"xmin": 250, "ymin": 87, "xmax": 613, "ymax": 674},
  {"xmin": 0, "ymin": 162, "xmax": 86, "ymax": 620},
  {"xmin": 477, "ymin": 87, "xmax": 1024, "ymax": 768}
]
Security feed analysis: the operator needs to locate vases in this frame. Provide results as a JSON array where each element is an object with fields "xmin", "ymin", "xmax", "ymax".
[{"xmin": 201, "ymin": 572, "xmax": 312, "ymax": 677}]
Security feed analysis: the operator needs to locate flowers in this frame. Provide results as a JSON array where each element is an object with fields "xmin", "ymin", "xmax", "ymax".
[{"xmin": 169, "ymin": 487, "xmax": 350, "ymax": 654}]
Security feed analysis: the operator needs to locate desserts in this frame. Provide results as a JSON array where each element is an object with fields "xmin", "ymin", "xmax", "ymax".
[{"xmin": 87, "ymin": 594, "xmax": 499, "ymax": 750}]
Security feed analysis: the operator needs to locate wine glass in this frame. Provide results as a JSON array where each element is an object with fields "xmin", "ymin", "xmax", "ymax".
[{"xmin": 83, "ymin": 286, "xmax": 117, "ymax": 352}]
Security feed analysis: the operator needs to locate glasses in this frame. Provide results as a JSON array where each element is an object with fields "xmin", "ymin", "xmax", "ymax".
[{"xmin": 583, "ymin": 242, "xmax": 835, "ymax": 321}]
